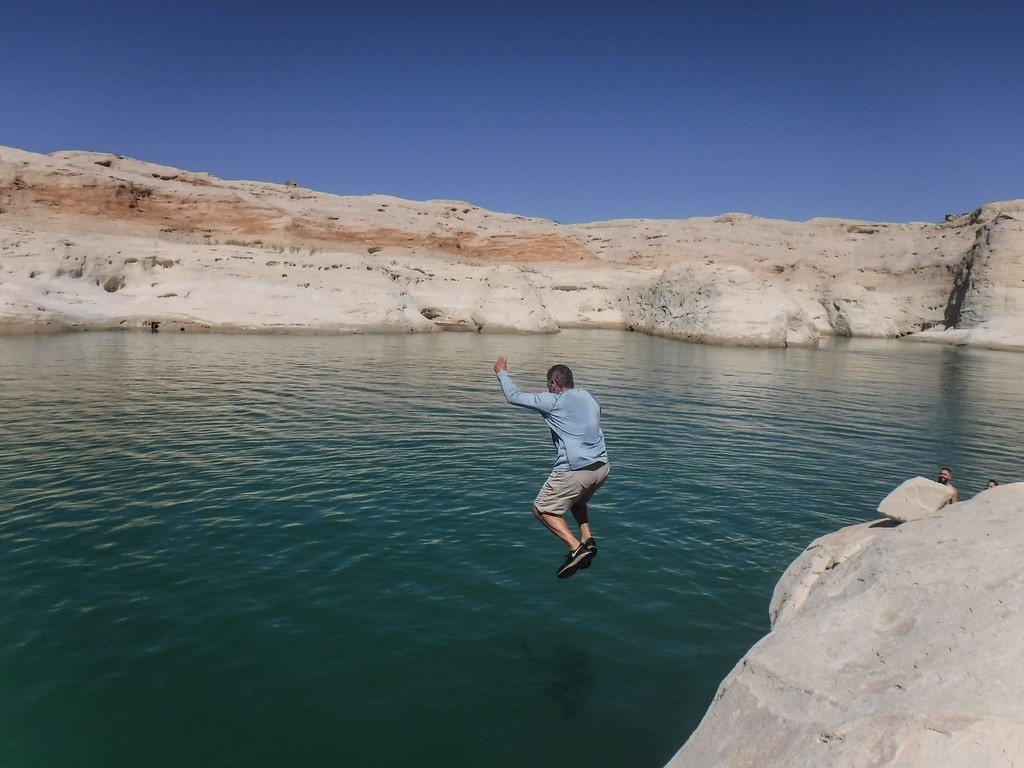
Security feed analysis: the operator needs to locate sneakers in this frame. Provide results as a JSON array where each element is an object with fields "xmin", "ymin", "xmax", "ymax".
[
  {"xmin": 557, "ymin": 543, "xmax": 593, "ymax": 578},
  {"xmin": 577, "ymin": 538, "xmax": 597, "ymax": 569}
]
